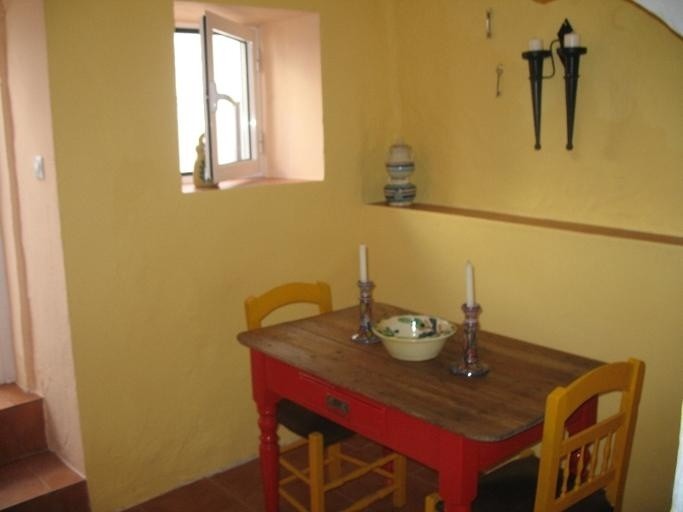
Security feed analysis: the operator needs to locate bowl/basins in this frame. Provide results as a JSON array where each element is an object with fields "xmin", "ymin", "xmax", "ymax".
[{"xmin": 371, "ymin": 313, "xmax": 458, "ymax": 362}]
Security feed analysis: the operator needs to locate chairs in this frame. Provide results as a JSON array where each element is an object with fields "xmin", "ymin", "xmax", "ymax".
[
  {"xmin": 425, "ymin": 358, "xmax": 645, "ymax": 512},
  {"xmin": 244, "ymin": 280, "xmax": 407, "ymax": 512}
]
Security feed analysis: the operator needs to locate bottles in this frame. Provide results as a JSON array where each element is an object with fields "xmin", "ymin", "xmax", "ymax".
[{"xmin": 193, "ymin": 134, "xmax": 218, "ymax": 188}]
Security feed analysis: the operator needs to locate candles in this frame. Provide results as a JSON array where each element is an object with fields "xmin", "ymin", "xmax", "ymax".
[
  {"xmin": 564, "ymin": 33, "xmax": 580, "ymax": 48},
  {"xmin": 528, "ymin": 38, "xmax": 543, "ymax": 51},
  {"xmin": 466, "ymin": 263, "xmax": 474, "ymax": 308},
  {"xmin": 359, "ymin": 245, "xmax": 367, "ymax": 283}
]
